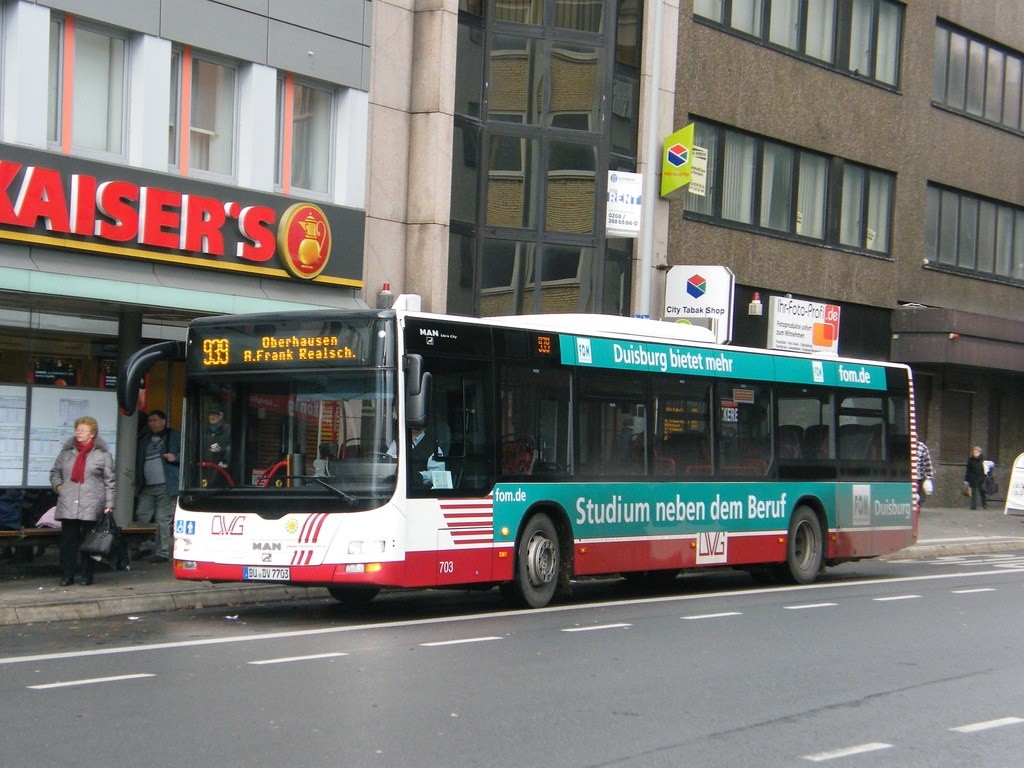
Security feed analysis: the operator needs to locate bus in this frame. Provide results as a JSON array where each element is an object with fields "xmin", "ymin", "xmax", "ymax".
[{"xmin": 117, "ymin": 293, "xmax": 922, "ymax": 610}]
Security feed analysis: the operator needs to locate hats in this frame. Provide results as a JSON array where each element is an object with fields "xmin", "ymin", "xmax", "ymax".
[
  {"xmin": 973, "ymin": 446, "xmax": 982, "ymax": 453},
  {"xmin": 208, "ymin": 405, "xmax": 224, "ymax": 415}
]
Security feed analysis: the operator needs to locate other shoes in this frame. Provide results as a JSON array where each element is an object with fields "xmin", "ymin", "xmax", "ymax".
[
  {"xmin": 80, "ymin": 574, "xmax": 93, "ymax": 587},
  {"xmin": 59, "ymin": 576, "xmax": 74, "ymax": 586},
  {"xmin": 150, "ymin": 555, "xmax": 168, "ymax": 564}
]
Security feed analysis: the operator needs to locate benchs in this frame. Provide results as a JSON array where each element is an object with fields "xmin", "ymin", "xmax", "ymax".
[{"xmin": 0, "ymin": 527, "xmax": 159, "ymax": 578}]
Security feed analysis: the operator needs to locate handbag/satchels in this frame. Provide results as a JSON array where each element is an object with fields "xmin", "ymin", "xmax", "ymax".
[
  {"xmin": 963, "ymin": 483, "xmax": 972, "ymax": 498},
  {"xmin": 981, "ymin": 476, "xmax": 998, "ymax": 495},
  {"xmin": 80, "ymin": 514, "xmax": 130, "ymax": 569},
  {"xmin": 922, "ymin": 476, "xmax": 934, "ymax": 496}
]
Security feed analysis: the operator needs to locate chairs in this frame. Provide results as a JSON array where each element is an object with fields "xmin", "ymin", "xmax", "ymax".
[
  {"xmin": 346, "ymin": 445, "xmax": 362, "ymax": 458},
  {"xmin": 497, "ymin": 434, "xmax": 539, "ymax": 478},
  {"xmin": 630, "ymin": 424, "xmax": 881, "ymax": 477}
]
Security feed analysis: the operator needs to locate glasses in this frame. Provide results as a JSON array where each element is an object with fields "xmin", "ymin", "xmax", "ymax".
[{"xmin": 75, "ymin": 428, "xmax": 92, "ymax": 433}]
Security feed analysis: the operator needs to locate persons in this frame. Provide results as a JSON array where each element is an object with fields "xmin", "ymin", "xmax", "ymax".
[
  {"xmin": 963, "ymin": 445, "xmax": 992, "ymax": 509},
  {"xmin": 917, "ymin": 434, "xmax": 935, "ymax": 514},
  {"xmin": 134, "ymin": 410, "xmax": 181, "ymax": 563},
  {"xmin": 49, "ymin": 416, "xmax": 118, "ymax": 586},
  {"xmin": 382, "ymin": 428, "xmax": 445, "ymax": 485},
  {"xmin": 205, "ymin": 404, "xmax": 232, "ymax": 485}
]
